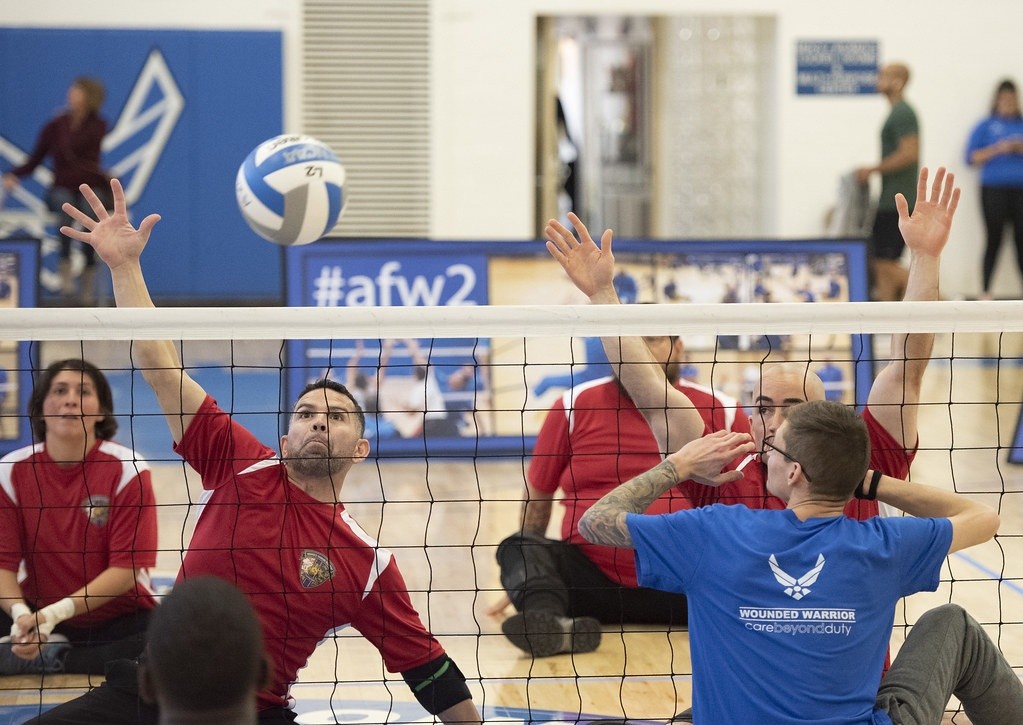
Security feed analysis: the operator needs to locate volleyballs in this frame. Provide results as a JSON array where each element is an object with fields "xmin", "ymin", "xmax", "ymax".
[{"xmin": 234, "ymin": 131, "xmax": 348, "ymax": 248}]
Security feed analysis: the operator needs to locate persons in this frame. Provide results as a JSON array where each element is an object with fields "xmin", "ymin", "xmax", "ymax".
[
  {"xmin": 19, "ymin": 179, "xmax": 483, "ymax": 724},
  {"xmin": 340, "ymin": 339, "xmax": 478, "ymax": 435},
  {"xmin": 0, "ymin": 359, "xmax": 162, "ymax": 675},
  {"xmin": 5, "ymin": 79, "xmax": 109, "ymax": 300},
  {"xmin": 577, "ymin": 400, "xmax": 1023, "ymax": 725},
  {"xmin": 855, "ymin": 64, "xmax": 920, "ymax": 301},
  {"xmin": 488, "ymin": 302, "xmax": 758, "ymax": 655},
  {"xmin": 545, "ymin": 168, "xmax": 960, "ymax": 677},
  {"xmin": 616, "ymin": 264, "xmax": 844, "ymax": 400},
  {"xmin": 966, "ymin": 80, "xmax": 1023, "ymax": 301}
]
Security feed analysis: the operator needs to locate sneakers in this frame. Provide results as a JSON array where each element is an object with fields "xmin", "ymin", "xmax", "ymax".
[{"xmin": 0, "ymin": 633, "xmax": 72, "ymax": 675}]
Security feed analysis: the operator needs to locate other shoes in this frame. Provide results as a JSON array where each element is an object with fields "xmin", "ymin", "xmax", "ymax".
[{"xmin": 501, "ymin": 610, "xmax": 601, "ymax": 657}]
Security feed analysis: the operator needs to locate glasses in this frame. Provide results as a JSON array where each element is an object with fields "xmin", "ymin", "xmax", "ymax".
[{"xmin": 762, "ymin": 435, "xmax": 812, "ymax": 483}]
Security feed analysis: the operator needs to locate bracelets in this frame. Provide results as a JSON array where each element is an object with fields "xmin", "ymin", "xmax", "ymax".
[{"xmin": 854, "ymin": 470, "xmax": 881, "ymax": 500}]
